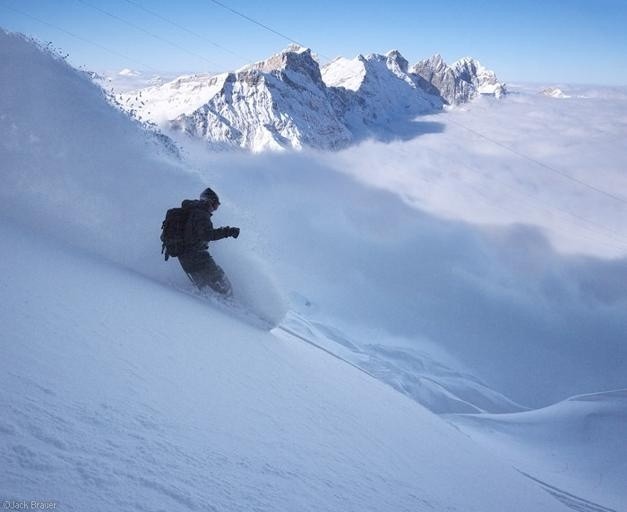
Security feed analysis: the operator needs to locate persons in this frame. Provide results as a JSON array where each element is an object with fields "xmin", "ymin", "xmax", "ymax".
[{"xmin": 177, "ymin": 187, "xmax": 240, "ymax": 301}]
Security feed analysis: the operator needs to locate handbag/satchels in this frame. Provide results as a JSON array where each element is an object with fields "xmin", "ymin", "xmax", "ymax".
[{"xmin": 161, "ymin": 208, "xmax": 185, "ymax": 261}]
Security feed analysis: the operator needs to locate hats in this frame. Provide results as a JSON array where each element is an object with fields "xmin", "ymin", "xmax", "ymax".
[{"xmin": 201, "ymin": 188, "xmax": 220, "ymax": 205}]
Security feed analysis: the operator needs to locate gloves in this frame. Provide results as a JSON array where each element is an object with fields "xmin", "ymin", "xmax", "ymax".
[{"xmin": 224, "ymin": 226, "xmax": 240, "ymax": 238}]
